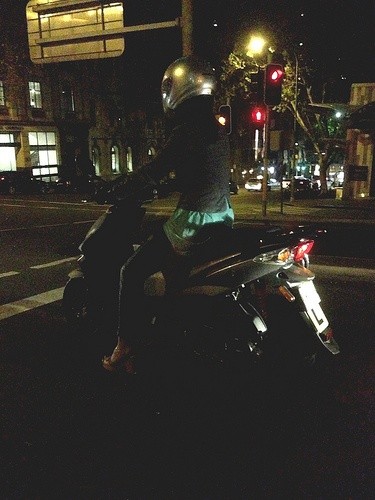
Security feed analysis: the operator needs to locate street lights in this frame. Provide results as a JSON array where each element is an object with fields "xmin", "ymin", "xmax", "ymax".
[{"xmin": 247, "ymin": 35, "xmax": 299, "ymax": 132}]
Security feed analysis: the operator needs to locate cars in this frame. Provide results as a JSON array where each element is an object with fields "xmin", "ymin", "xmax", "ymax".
[
  {"xmin": 0, "ymin": 170, "xmax": 50, "ymax": 196},
  {"xmin": 228, "ymin": 180, "xmax": 240, "ymax": 194},
  {"xmin": 244, "ymin": 178, "xmax": 272, "ymax": 192}
]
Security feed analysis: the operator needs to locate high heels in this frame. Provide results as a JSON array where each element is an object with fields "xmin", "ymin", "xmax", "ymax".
[{"xmin": 100, "ymin": 342, "xmax": 136, "ymax": 376}]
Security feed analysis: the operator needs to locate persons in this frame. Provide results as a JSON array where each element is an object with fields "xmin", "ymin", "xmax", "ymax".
[
  {"xmin": 72, "ymin": 148, "xmax": 88, "ymax": 202},
  {"xmin": 100, "ymin": 52, "xmax": 238, "ymax": 378}
]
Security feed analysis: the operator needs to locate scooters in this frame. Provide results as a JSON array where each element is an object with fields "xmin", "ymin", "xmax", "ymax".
[{"xmin": 53, "ymin": 180, "xmax": 340, "ymax": 386}]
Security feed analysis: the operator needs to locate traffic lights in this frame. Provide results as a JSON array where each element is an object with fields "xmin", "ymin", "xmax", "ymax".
[
  {"xmin": 218, "ymin": 115, "xmax": 227, "ymax": 126},
  {"xmin": 269, "ymin": 68, "xmax": 284, "ymax": 84},
  {"xmin": 251, "ymin": 109, "xmax": 265, "ymax": 123}
]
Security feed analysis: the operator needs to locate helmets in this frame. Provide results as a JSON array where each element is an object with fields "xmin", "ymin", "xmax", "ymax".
[{"xmin": 160, "ymin": 56, "xmax": 217, "ymax": 113}]
